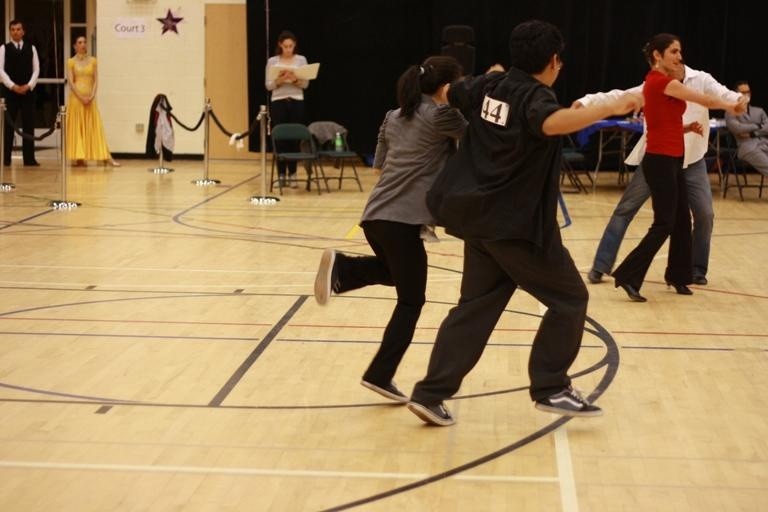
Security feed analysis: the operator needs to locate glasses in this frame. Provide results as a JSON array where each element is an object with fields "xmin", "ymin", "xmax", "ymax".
[{"xmin": 741, "ymin": 90, "xmax": 752, "ymax": 94}]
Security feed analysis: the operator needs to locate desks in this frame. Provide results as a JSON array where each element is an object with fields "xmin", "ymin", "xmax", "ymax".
[
  {"xmin": 591, "ymin": 119, "xmax": 642, "ymax": 188},
  {"xmin": 704, "ymin": 118, "xmax": 726, "ymax": 188}
]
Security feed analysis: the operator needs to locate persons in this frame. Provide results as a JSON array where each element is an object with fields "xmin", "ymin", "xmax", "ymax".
[
  {"xmin": 407, "ymin": 20, "xmax": 644, "ymax": 427},
  {"xmin": 315, "ymin": 56, "xmax": 470, "ymax": 403},
  {"xmin": 0, "ymin": 20, "xmax": 40, "ymax": 167},
  {"xmin": 265, "ymin": 31, "xmax": 308, "ymax": 189},
  {"xmin": 65, "ymin": 34, "xmax": 121, "ymax": 168},
  {"xmin": 726, "ymin": 80, "xmax": 768, "ymax": 178},
  {"xmin": 569, "ymin": 43, "xmax": 750, "ymax": 285},
  {"xmin": 611, "ymin": 32, "xmax": 746, "ymax": 301}
]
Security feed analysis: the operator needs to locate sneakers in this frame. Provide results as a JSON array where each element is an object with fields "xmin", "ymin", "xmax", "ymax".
[
  {"xmin": 361, "ymin": 372, "xmax": 408, "ymax": 403},
  {"xmin": 288, "ymin": 175, "xmax": 298, "ymax": 188},
  {"xmin": 408, "ymin": 397, "xmax": 456, "ymax": 426},
  {"xmin": 273, "ymin": 175, "xmax": 286, "ymax": 187},
  {"xmin": 315, "ymin": 246, "xmax": 340, "ymax": 307},
  {"xmin": 533, "ymin": 384, "xmax": 601, "ymax": 420}
]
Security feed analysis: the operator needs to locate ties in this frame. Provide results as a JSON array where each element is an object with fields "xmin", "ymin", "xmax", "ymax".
[{"xmin": 17, "ymin": 43, "xmax": 21, "ymax": 53}]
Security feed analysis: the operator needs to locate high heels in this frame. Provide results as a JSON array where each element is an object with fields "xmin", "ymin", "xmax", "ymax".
[
  {"xmin": 99, "ymin": 158, "xmax": 122, "ymax": 170},
  {"xmin": 663, "ymin": 268, "xmax": 693, "ymax": 297},
  {"xmin": 613, "ymin": 271, "xmax": 647, "ymax": 302}
]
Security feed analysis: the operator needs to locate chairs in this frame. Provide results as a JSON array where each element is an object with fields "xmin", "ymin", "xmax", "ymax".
[
  {"xmin": 270, "ymin": 124, "xmax": 321, "ymax": 195},
  {"xmin": 561, "ymin": 141, "xmax": 594, "ymax": 184},
  {"xmin": 307, "ymin": 122, "xmax": 364, "ymax": 192},
  {"xmin": 723, "ymin": 128, "xmax": 764, "ymax": 201},
  {"xmin": 557, "ymin": 153, "xmax": 589, "ymax": 194}
]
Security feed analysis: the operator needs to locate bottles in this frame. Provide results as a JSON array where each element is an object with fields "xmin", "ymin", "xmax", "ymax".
[{"xmin": 336, "ymin": 133, "xmax": 346, "ymax": 152}]
